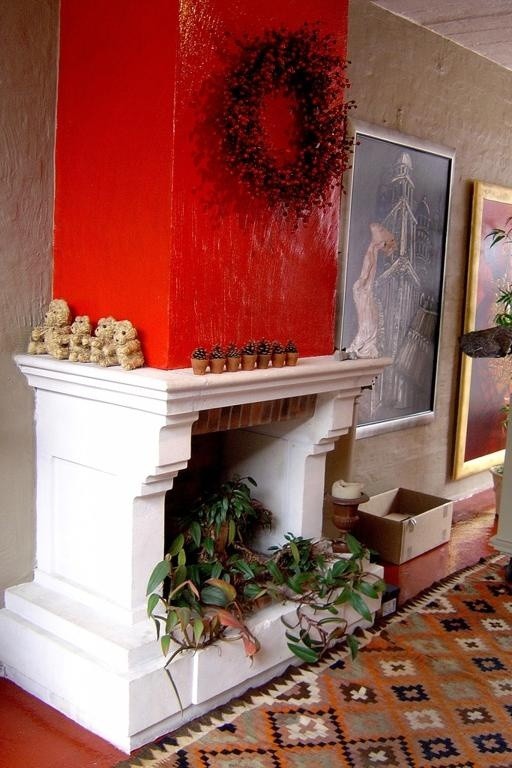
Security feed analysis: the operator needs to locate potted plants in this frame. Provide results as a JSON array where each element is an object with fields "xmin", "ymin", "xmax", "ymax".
[
  {"xmin": 190, "ymin": 340, "xmax": 299, "ymax": 374},
  {"xmin": 482, "ymin": 213, "xmax": 512, "ymax": 517}
]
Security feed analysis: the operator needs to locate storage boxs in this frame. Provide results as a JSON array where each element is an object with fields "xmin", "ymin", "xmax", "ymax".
[{"xmin": 357, "ymin": 487, "xmax": 456, "ymax": 564}]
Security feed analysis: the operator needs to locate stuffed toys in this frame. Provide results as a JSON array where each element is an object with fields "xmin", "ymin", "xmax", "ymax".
[
  {"xmin": 104, "ymin": 318, "xmax": 144, "ymax": 369},
  {"xmin": 56, "ymin": 315, "xmax": 91, "ymax": 363},
  {"xmin": 88, "ymin": 314, "xmax": 119, "ymax": 367},
  {"xmin": 27, "ymin": 297, "xmax": 69, "ymax": 361}
]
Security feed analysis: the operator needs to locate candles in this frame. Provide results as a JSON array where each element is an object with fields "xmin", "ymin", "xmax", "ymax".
[{"xmin": 332, "ymin": 479, "xmax": 361, "ymax": 499}]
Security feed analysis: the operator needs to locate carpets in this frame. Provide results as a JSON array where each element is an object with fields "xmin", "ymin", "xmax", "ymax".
[{"xmin": 122, "ymin": 553, "xmax": 512, "ymax": 768}]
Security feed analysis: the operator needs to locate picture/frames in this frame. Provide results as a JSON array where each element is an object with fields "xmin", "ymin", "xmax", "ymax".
[
  {"xmin": 214, "ymin": 19, "xmax": 359, "ymax": 223},
  {"xmin": 451, "ymin": 179, "xmax": 511, "ymax": 480},
  {"xmin": 337, "ymin": 116, "xmax": 456, "ymax": 442}
]
